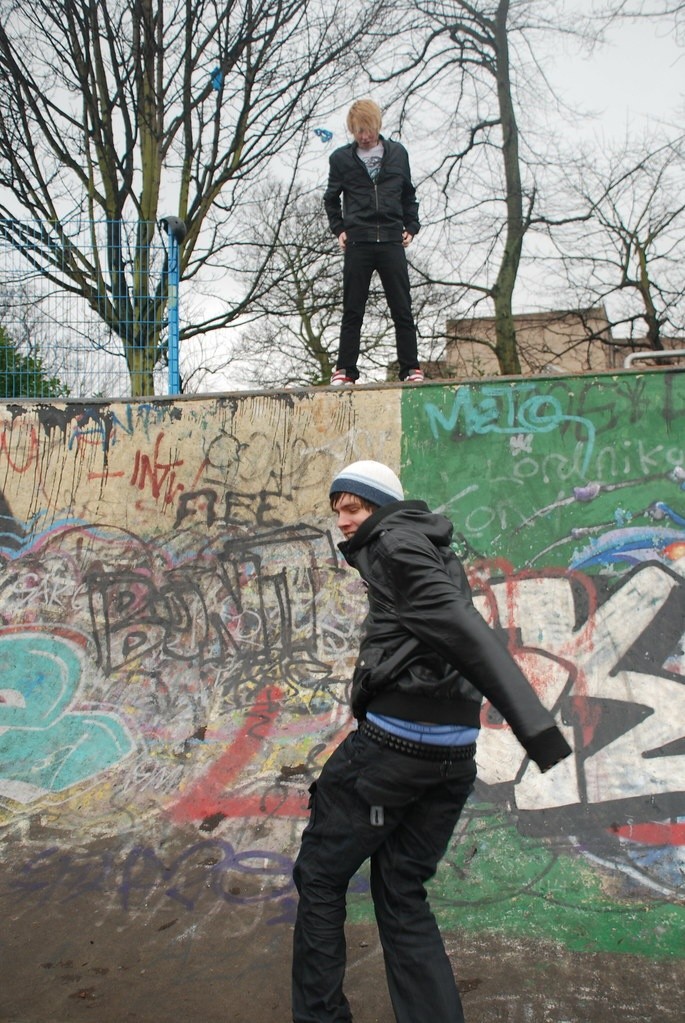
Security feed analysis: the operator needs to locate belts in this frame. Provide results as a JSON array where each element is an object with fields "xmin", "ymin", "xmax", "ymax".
[{"xmin": 359, "ymin": 721, "xmax": 477, "ymax": 760}]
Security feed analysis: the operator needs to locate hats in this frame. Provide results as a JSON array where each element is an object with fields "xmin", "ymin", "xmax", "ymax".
[{"xmin": 327, "ymin": 459, "xmax": 403, "ymax": 506}]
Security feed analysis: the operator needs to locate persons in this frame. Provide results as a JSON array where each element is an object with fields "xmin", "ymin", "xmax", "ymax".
[
  {"xmin": 292, "ymin": 460, "xmax": 573, "ymax": 1023},
  {"xmin": 324, "ymin": 99, "xmax": 425, "ymax": 386}
]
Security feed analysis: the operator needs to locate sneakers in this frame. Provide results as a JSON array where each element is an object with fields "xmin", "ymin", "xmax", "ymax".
[
  {"xmin": 403, "ymin": 369, "xmax": 424, "ymax": 381},
  {"xmin": 328, "ymin": 369, "xmax": 355, "ymax": 385}
]
